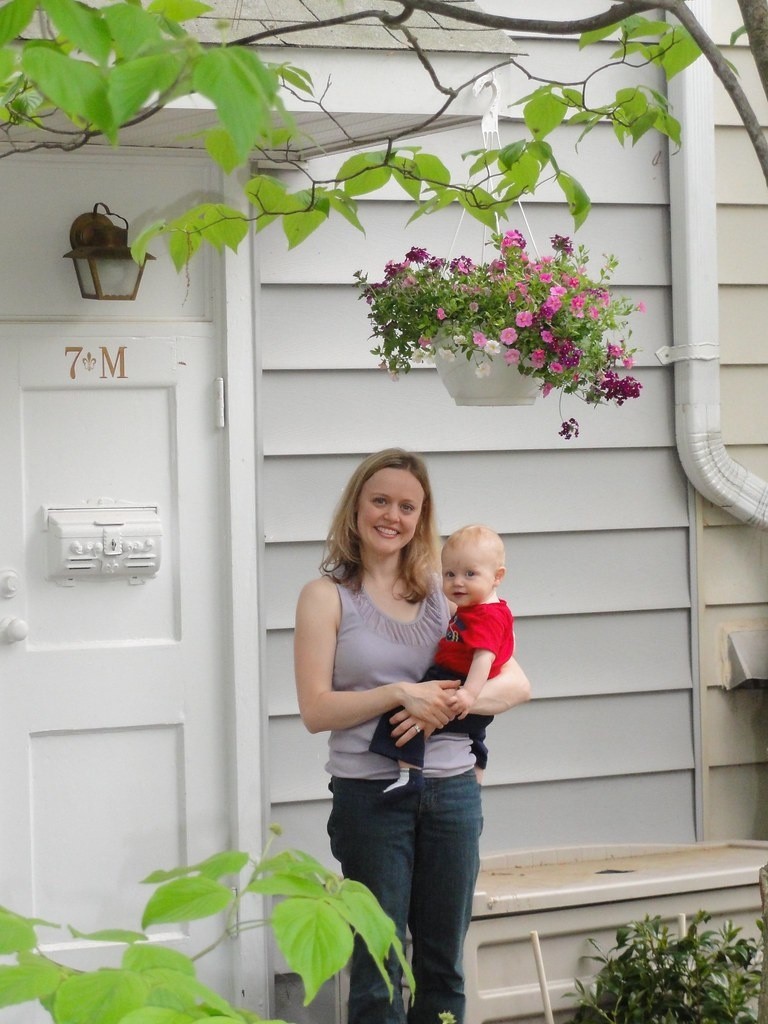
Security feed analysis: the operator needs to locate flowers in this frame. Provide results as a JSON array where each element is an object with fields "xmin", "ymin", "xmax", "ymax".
[{"xmin": 351, "ymin": 228, "xmax": 648, "ymax": 438}]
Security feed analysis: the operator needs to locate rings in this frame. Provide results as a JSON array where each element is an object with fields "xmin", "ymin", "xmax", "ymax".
[{"xmin": 414, "ymin": 725, "xmax": 421, "ymax": 733}]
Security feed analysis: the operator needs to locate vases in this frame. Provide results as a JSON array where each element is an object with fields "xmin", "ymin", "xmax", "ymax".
[{"xmin": 419, "ymin": 318, "xmax": 540, "ymax": 408}]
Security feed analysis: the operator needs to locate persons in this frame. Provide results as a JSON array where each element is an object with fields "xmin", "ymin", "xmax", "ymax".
[
  {"xmin": 293, "ymin": 448, "xmax": 531, "ymax": 1024},
  {"xmin": 369, "ymin": 525, "xmax": 515, "ymax": 801}
]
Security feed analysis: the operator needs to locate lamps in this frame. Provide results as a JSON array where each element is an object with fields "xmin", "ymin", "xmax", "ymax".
[{"xmin": 62, "ymin": 202, "xmax": 160, "ymax": 303}]
